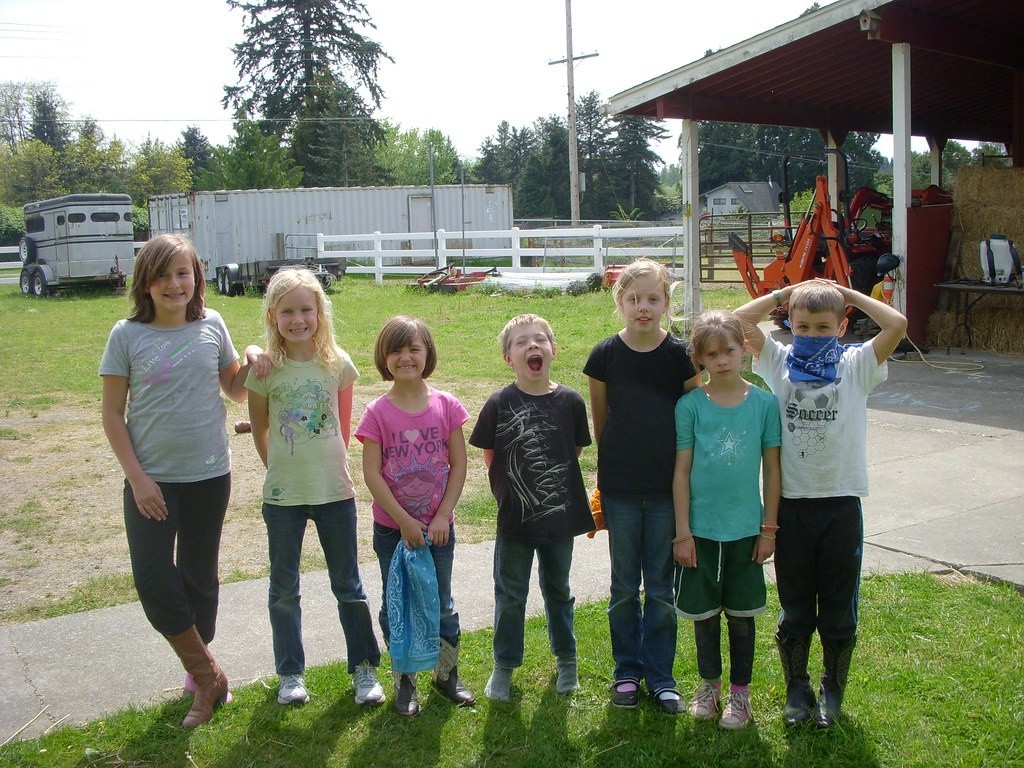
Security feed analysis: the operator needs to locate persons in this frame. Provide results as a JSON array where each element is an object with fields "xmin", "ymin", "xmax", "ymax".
[
  {"xmin": 583, "ymin": 261, "xmax": 703, "ymax": 717},
  {"xmin": 352, "ymin": 315, "xmax": 475, "ymax": 715},
  {"xmin": 672, "ymin": 310, "xmax": 782, "ymax": 732},
  {"xmin": 98, "ymin": 233, "xmax": 272, "ymax": 729},
  {"xmin": 732, "ymin": 278, "xmax": 908, "ymax": 729},
  {"xmin": 244, "ymin": 265, "xmax": 386, "ymax": 706},
  {"xmin": 467, "ymin": 314, "xmax": 595, "ymax": 704}
]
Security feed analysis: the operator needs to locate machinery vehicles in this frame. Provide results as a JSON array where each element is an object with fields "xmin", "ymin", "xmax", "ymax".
[{"xmin": 728, "ymin": 173, "xmax": 921, "ymax": 335}]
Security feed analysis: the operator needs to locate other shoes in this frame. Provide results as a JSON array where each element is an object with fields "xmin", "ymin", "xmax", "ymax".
[
  {"xmin": 485, "ymin": 665, "xmax": 512, "ymax": 702},
  {"xmin": 610, "ymin": 679, "xmax": 640, "ymax": 708},
  {"xmin": 650, "ymin": 686, "xmax": 687, "ymax": 715},
  {"xmin": 555, "ymin": 659, "xmax": 580, "ymax": 693}
]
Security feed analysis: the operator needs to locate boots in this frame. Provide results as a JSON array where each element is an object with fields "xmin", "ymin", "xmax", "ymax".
[
  {"xmin": 167, "ymin": 625, "xmax": 228, "ymax": 726},
  {"xmin": 430, "ymin": 612, "xmax": 475, "ymax": 706},
  {"xmin": 777, "ymin": 636, "xmax": 814, "ymax": 726},
  {"xmin": 378, "ymin": 612, "xmax": 421, "ymax": 716},
  {"xmin": 814, "ymin": 638, "xmax": 856, "ymax": 726}
]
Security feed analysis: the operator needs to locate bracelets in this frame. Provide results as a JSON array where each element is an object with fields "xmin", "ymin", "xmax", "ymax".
[
  {"xmin": 760, "ymin": 525, "xmax": 780, "ymax": 539},
  {"xmin": 773, "ymin": 290, "xmax": 783, "ymax": 309},
  {"xmin": 674, "ymin": 534, "xmax": 693, "ymax": 544}
]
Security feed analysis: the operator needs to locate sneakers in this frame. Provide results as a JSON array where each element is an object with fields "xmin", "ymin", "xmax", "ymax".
[
  {"xmin": 262, "ymin": 673, "xmax": 317, "ymax": 705},
  {"xmin": 689, "ymin": 682, "xmax": 722, "ymax": 720},
  {"xmin": 718, "ymin": 692, "xmax": 751, "ymax": 729},
  {"xmin": 352, "ymin": 659, "xmax": 386, "ymax": 704}
]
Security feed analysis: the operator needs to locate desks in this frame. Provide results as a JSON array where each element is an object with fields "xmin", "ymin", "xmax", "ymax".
[{"xmin": 933, "ymin": 278, "xmax": 1024, "ymax": 355}]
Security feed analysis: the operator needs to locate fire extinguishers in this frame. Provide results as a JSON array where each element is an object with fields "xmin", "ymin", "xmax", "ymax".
[{"xmin": 882, "ymin": 270, "xmax": 893, "ymax": 305}]
